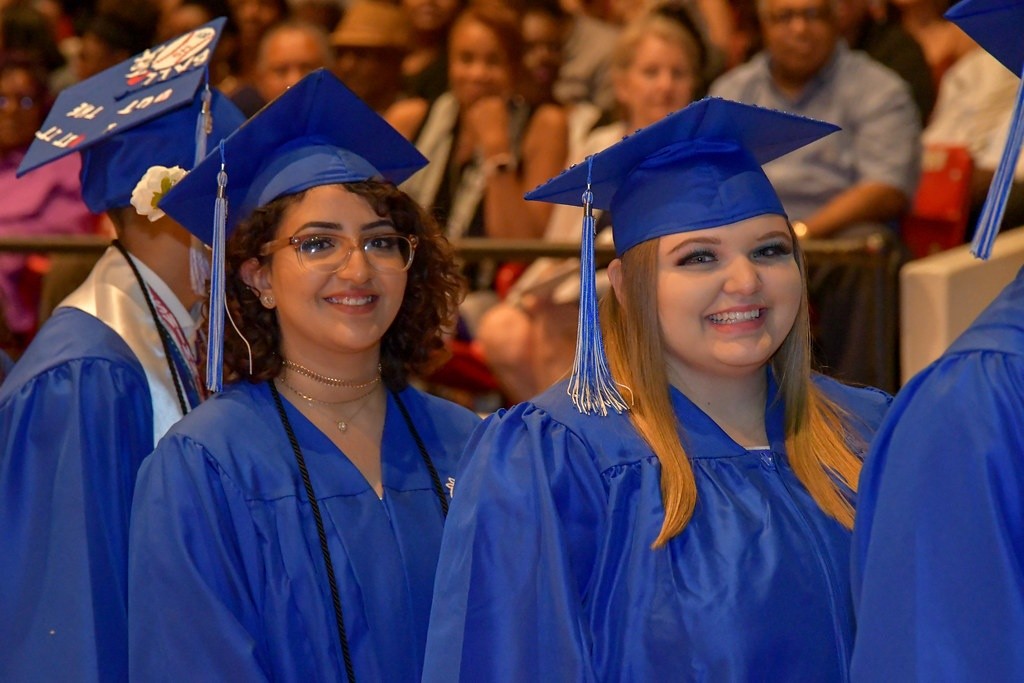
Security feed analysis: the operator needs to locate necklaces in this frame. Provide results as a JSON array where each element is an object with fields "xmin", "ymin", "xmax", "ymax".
[{"xmin": 274, "ymin": 359, "xmax": 382, "ymax": 433}]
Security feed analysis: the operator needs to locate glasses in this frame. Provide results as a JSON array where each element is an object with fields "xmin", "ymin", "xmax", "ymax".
[{"xmin": 258, "ymin": 234, "xmax": 419, "ymax": 273}]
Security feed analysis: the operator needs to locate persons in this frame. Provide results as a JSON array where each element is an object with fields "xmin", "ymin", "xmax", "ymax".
[
  {"xmin": 851, "ymin": 0, "xmax": 1024, "ymax": 683},
  {"xmin": 0, "ymin": 18, "xmax": 248, "ymax": 683},
  {"xmin": 0, "ymin": 0, "xmax": 1024, "ymax": 418},
  {"xmin": 420, "ymin": 95, "xmax": 896, "ymax": 682},
  {"xmin": 127, "ymin": 66, "xmax": 483, "ymax": 683}
]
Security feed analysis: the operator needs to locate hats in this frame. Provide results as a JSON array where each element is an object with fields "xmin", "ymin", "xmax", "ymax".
[
  {"xmin": 14, "ymin": 16, "xmax": 248, "ymax": 295},
  {"xmin": 944, "ymin": 0, "xmax": 1024, "ymax": 260},
  {"xmin": 155, "ymin": 67, "xmax": 431, "ymax": 394},
  {"xmin": 330, "ymin": 4, "xmax": 410, "ymax": 46},
  {"xmin": 523, "ymin": 96, "xmax": 841, "ymax": 417}
]
